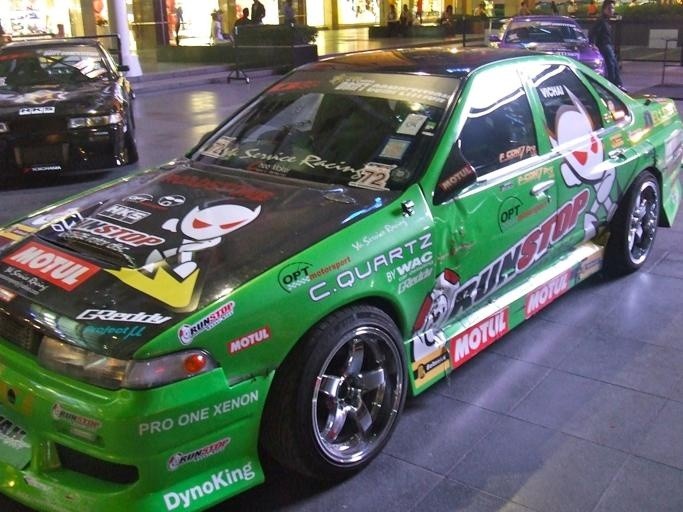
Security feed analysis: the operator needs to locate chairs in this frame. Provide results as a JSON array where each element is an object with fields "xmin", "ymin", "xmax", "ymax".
[
  {"xmin": 551, "ymin": 29, "xmax": 563, "ymax": 41},
  {"xmin": 512, "ymin": 27, "xmax": 529, "ymax": 39}
]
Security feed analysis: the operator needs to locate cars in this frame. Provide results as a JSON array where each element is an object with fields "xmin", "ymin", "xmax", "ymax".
[
  {"xmin": 0, "ymin": 39, "xmax": 138, "ymax": 184},
  {"xmin": 489, "ymin": 15, "xmax": 607, "ymax": 94},
  {"xmin": 0, "ymin": 46, "xmax": 683, "ymax": 508}
]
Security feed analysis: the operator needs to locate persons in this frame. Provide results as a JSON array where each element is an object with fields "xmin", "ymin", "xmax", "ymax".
[
  {"xmin": 387, "ymin": 0, "xmax": 598, "ymax": 38},
  {"xmin": 233, "ymin": 8, "xmax": 251, "ymax": 26},
  {"xmin": 253, "ymin": 1, "xmax": 266, "ymax": 20},
  {"xmin": 284, "ymin": 1, "xmax": 297, "ymax": 23},
  {"xmin": 591, "ymin": 0, "xmax": 629, "ymax": 92}
]
